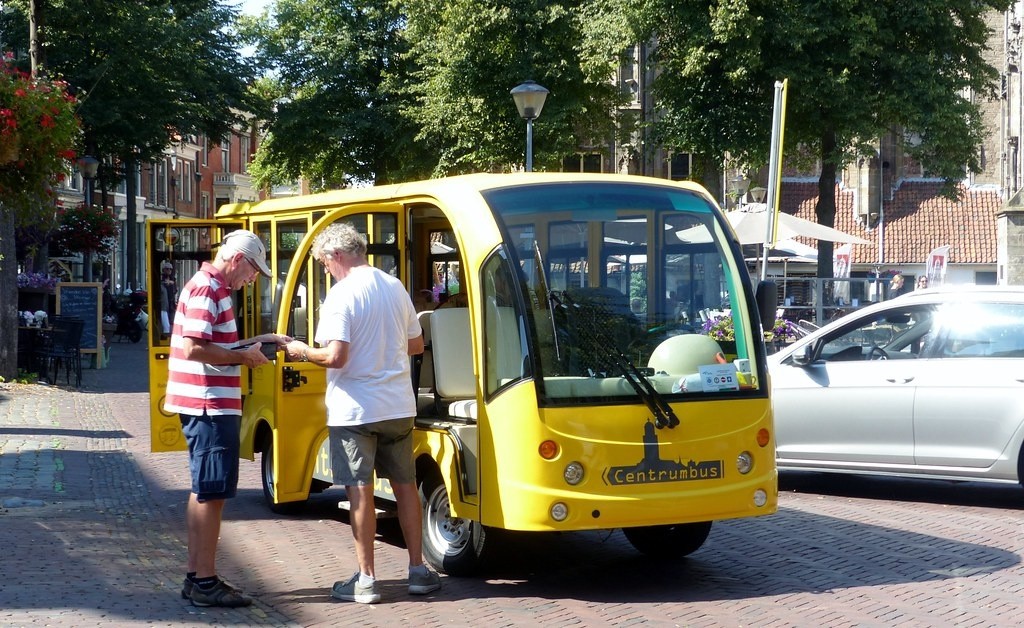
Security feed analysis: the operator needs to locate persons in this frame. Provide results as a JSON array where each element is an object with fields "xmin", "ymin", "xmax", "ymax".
[
  {"xmin": 281, "ymin": 222, "xmax": 441, "ymax": 605},
  {"xmin": 911, "ymin": 275, "xmax": 928, "ymax": 321},
  {"xmin": 162, "ymin": 262, "xmax": 177, "ymax": 332},
  {"xmin": 162, "ymin": 229, "xmax": 293, "ymax": 605},
  {"xmin": 885, "ymin": 274, "xmax": 910, "ymax": 323}
]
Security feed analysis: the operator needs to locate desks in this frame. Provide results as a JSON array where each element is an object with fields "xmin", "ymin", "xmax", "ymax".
[{"xmin": 19, "ymin": 326, "xmax": 67, "ymax": 372}]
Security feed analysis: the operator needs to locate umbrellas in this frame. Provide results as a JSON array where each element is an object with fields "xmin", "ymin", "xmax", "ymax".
[
  {"xmin": 675, "ymin": 202, "xmax": 875, "ymax": 286},
  {"xmin": 764, "ymin": 238, "xmax": 844, "ymax": 300},
  {"xmin": 520, "ymin": 216, "xmax": 673, "ymax": 290}
]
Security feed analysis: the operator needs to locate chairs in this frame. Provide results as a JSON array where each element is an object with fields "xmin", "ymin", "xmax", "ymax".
[
  {"xmin": 293, "ymin": 307, "xmax": 307, "ymax": 336},
  {"xmin": 404, "ymin": 286, "xmax": 642, "ymax": 422},
  {"xmin": 19, "ymin": 314, "xmax": 85, "ymax": 389}
]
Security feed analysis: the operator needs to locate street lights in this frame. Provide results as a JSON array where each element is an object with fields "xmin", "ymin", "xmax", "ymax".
[
  {"xmin": 732, "ymin": 173, "xmax": 767, "ymax": 285},
  {"xmin": 78, "ymin": 153, "xmax": 100, "ymax": 282},
  {"xmin": 510, "ymin": 80, "xmax": 551, "ymax": 171}
]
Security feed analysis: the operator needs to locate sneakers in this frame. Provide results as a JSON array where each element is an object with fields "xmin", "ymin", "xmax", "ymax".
[
  {"xmin": 189, "ymin": 579, "xmax": 252, "ymax": 607},
  {"xmin": 331, "ymin": 571, "xmax": 381, "ymax": 604},
  {"xmin": 182, "ymin": 577, "xmax": 243, "ymax": 600},
  {"xmin": 408, "ymin": 567, "xmax": 441, "ymax": 594}
]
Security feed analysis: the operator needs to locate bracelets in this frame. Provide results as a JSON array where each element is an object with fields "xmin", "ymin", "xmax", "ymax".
[{"xmin": 302, "ymin": 346, "xmax": 310, "ymax": 362}]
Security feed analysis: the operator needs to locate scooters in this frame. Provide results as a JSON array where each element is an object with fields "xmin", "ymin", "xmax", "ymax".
[{"xmin": 101, "ymin": 278, "xmax": 149, "ymax": 344}]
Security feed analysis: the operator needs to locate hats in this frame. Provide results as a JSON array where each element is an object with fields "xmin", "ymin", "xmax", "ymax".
[{"xmin": 222, "ymin": 229, "xmax": 273, "ymax": 278}]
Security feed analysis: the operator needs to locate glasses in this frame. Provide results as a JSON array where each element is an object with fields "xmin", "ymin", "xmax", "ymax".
[
  {"xmin": 244, "ymin": 257, "xmax": 260, "ymax": 277},
  {"xmin": 318, "ymin": 253, "xmax": 327, "ymax": 268}
]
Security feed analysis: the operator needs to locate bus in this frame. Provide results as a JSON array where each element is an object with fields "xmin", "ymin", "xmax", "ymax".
[{"xmin": 142, "ymin": 169, "xmax": 779, "ymax": 579}]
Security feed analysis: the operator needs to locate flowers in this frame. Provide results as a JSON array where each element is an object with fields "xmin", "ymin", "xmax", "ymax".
[
  {"xmin": 867, "ymin": 269, "xmax": 901, "ymax": 277},
  {"xmin": 22, "ymin": 310, "xmax": 47, "ymax": 328}
]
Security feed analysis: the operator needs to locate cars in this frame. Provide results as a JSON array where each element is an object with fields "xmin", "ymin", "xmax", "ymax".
[{"xmin": 766, "ymin": 283, "xmax": 1023, "ymax": 493}]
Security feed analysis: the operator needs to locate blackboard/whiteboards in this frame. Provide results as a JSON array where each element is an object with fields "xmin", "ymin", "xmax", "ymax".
[{"xmin": 56, "ymin": 282, "xmax": 102, "ymax": 353}]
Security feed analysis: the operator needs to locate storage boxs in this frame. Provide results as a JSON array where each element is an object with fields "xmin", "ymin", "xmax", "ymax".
[{"xmin": 129, "ymin": 290, "xmax": 148, "ymax": 304}]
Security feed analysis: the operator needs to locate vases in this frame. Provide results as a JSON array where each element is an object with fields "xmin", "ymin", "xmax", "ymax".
[
  {"xmin": 882, "ymin": 274, "xmax": 893, "ymax": 283},
  {"xmin": 42, "ymin": 318, "xmax": 48, "ymax": 327},
  {"xmin": 868, "ymin": 274, "xmax": 875, "ymax": 283}
]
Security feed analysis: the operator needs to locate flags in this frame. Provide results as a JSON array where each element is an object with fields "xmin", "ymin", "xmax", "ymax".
[
  {"xmin": 834, "ymin": 242, "xmax": 852, "ymax": 305},
  {"xmin": 927, "ymin": 245, "xmax": 951, "ymax": 288}
]
